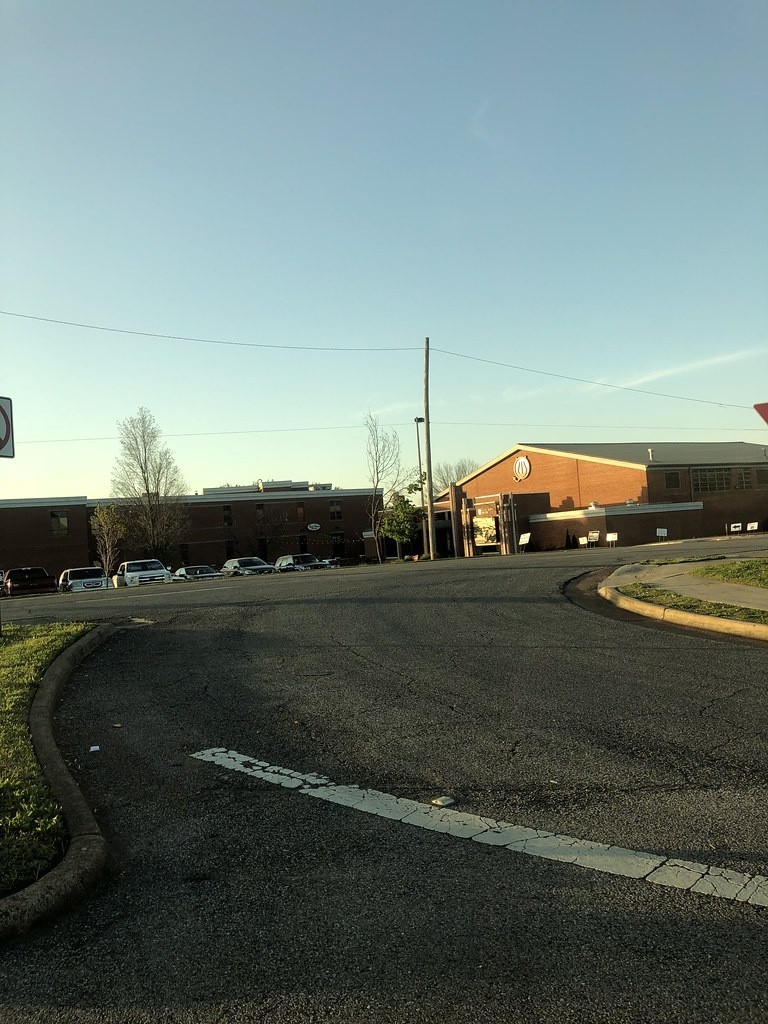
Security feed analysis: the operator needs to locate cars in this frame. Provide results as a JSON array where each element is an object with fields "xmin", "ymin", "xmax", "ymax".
[
  {"xmin": 172, "ymin": 565, "xmax": 225, "ymax": 582},
  {"xmin": 274, "ymin": 553, "xmax": 329, "ymax": 573},
  {"xmin": 321, "ymin": 559, "xmax": 341, "ymax": 569},
  {"xmin": 221, "ymin": 556, "xmax": 279, "ymax": 580},
  {"xmin": 112, "ymin": 559, "xmax": 174, "ymax": 588},
  {"xmin": 59, "ymin": 567, "xmax": 114, "ymax": 593}
]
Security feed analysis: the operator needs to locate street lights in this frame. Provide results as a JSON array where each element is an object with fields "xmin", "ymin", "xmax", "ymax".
[{"xmin": 414, "ymin": 416, "xmax": 432, "ymax": 559}]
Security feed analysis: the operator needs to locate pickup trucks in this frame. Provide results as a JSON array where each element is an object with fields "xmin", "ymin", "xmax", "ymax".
[{"xmin": 4, "ymin": 566, "xmax": 58, "ymax": 597}]
